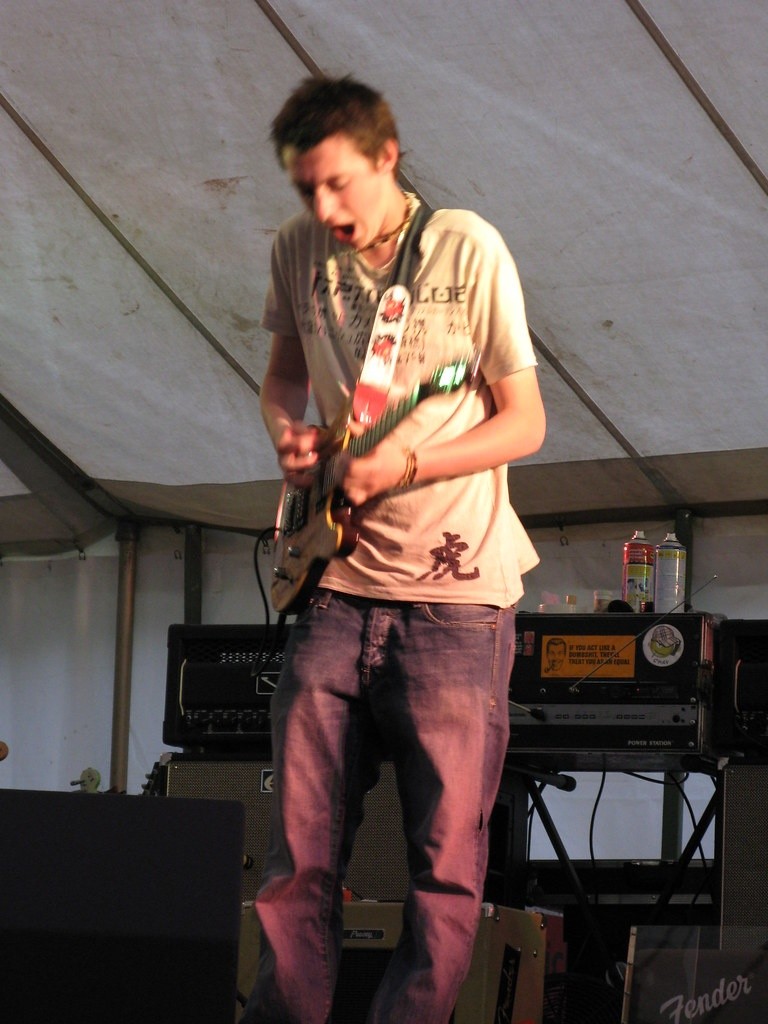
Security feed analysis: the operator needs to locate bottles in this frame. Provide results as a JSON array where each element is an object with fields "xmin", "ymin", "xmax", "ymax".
[
  {"xmin": 622, "ymin": 531, "xmax": 655, "ymax": 611},
  {"xmin": 654, "ymin": 533, "xmax": 685, "ymax": 614}
]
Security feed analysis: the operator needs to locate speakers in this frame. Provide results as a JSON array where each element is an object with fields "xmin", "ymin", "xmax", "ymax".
[
  {"xmin": 163, "ymin": 752, "xmax": 411, "ymax": 900},
  {"xmin": 0, "ymin": 788, "xmax": 247, "ymax": 1023},
  {"xmin": 713, "ymin": 763, "xmax": 768, "ymax": 952}
]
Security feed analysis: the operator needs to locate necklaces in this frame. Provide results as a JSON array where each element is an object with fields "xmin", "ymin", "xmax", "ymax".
[{"xmin": 361, "ymin": 191, "xmax": 412, "ymax": 252}]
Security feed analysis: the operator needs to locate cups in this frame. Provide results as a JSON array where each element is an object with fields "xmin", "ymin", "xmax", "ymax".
[{"xmin": 593, "ymin": 590, "xmax": 612, "ymax": 611}]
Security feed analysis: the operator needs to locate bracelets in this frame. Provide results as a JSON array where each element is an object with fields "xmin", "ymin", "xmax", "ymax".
[{"xmin": 395, "ymin": 448, "xmax": 418, "ymax": 494}]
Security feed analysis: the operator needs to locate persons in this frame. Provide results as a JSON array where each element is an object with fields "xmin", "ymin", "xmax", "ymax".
[{"xmin": 256, "ymin": 76, "xmax": 546, "ymax": 1024}]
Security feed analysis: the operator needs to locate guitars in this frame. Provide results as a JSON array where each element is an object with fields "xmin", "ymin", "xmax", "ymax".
[{"xmin": 270, "ymin": 359, "xmax": 472, "ymax": 615}]
